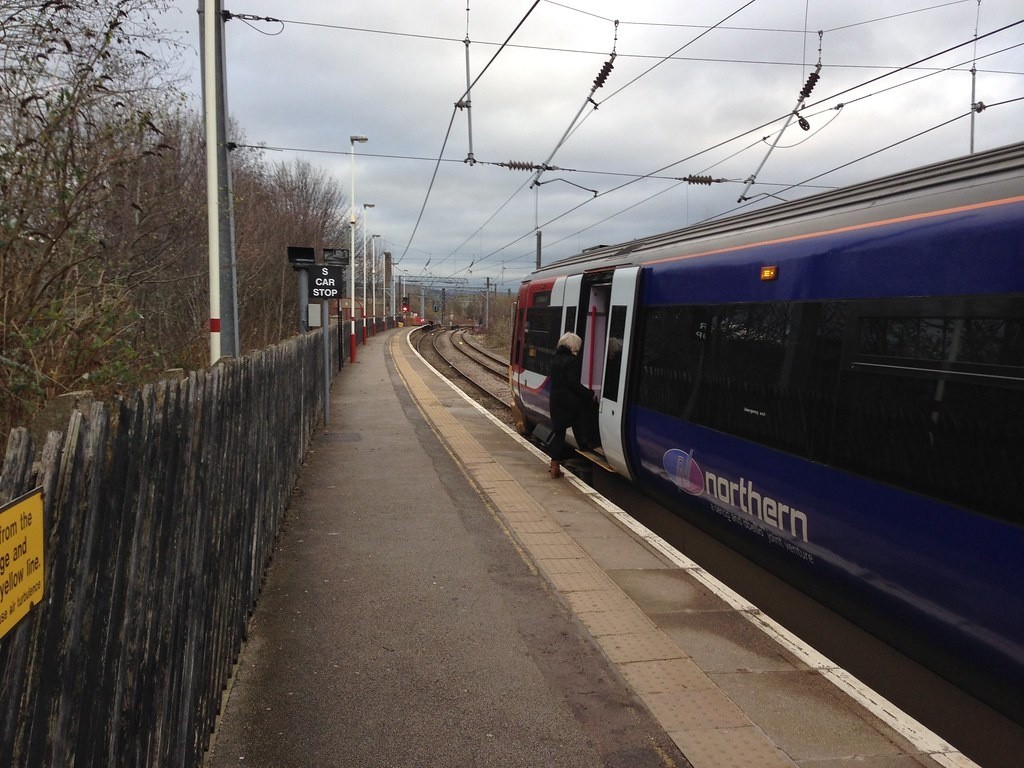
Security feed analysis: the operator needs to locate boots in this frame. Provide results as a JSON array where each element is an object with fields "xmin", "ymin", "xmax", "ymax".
[
  {"xmin": 587, "ymin": 440, "xmax": 601, "ymax": 450},
  {"xmin": 550, "ymin": 462, "xmax": 565, "ymax": 478}
]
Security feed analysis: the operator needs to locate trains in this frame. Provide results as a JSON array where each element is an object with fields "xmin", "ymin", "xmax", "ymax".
[{"xmin": 509, "ymin": 136, "xmax": 1024, "ymax": 724}]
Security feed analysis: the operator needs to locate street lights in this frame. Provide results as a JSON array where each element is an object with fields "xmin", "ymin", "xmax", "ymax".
[
  {"xmin": 349, "ymin": 135, "xmax": 368, "ymax": 364},
  {"xmin": 362, "ymin": 203, "xmax": 409, "ymax": 346}
]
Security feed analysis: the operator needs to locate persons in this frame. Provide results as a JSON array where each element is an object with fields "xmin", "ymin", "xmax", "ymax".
[
  {"xmin": 601, "ymin": 337, "xmax": 624, "ymax": 403},
  {"xmin": 549, "ymin": 331, "xmax": 602, "ymax": 479}
]
